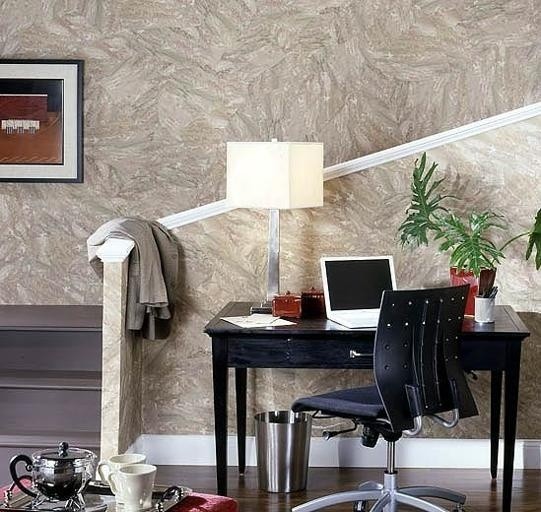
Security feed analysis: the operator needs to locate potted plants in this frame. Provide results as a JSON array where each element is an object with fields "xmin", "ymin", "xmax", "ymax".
[{"xmin": 395, "ymin": 151, "xmax": 541, "ymax": 318}]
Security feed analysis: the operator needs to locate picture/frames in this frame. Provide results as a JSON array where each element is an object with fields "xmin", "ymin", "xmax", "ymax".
[{"xmin": 0, "ymin": 59, "xmax": 85, "ymax": 183}]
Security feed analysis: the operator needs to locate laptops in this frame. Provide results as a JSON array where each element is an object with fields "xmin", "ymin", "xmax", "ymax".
[{"xmin": 320, "ymin": 255, "xmax": 397, "ymax": 329}]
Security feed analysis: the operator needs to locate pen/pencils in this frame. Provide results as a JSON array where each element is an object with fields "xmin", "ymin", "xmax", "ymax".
[{"xmin": 482, "ymin": 286, "xmax": 498, "ymax": 298}]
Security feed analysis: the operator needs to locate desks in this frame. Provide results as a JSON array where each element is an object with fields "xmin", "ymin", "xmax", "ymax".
[
  {"xmin": 204, "ymin": 301, "xmax": 531, "ymax": 511},
  {"xmin": 0, "ymin": 477, "xmax": 240, "ymax": 511}
]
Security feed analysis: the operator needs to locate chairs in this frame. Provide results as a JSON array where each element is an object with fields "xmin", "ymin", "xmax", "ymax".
[{"xmin": 288, "ymin": 283, "xmax": 467, "ymax": 511}]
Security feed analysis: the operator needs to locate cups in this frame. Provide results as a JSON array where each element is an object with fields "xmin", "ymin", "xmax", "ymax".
[
  {"xmin": 98, "ymin": 454, "xmax": 157, "ymax": 511},
  {"xmin": 473, "ymin": 295, "xmax": 495, "ymax": 323}
]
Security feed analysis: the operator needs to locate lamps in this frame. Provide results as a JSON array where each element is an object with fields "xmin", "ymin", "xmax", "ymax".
[{"xmin": 226, "ymin": 138, "xmax": 325, "ymax": 312}]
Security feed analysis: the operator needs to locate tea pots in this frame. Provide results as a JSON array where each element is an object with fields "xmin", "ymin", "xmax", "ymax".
[{"xmin": 8, "ymin": 442, "xmax": 99, "ymax": 510}]
